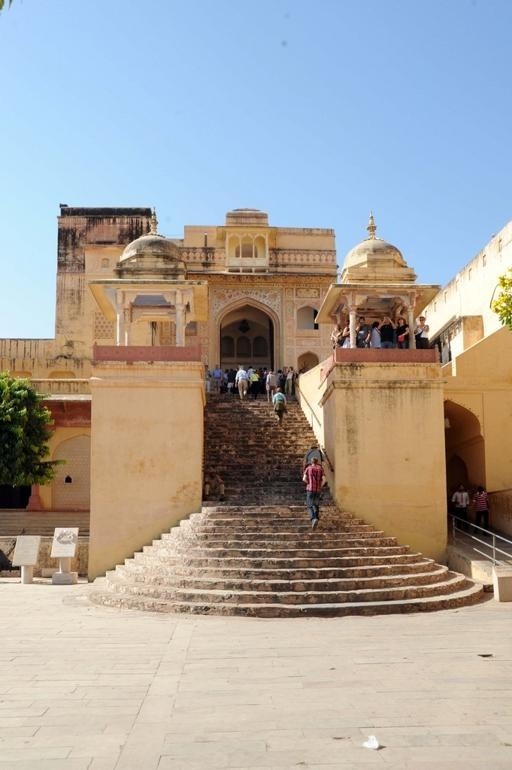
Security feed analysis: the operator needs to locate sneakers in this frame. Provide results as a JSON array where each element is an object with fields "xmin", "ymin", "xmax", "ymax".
[{"xmin": 311, "ymin": 518, "xmax": 319, "ymax": 531}]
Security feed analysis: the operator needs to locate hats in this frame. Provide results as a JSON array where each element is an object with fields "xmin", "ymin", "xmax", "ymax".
[{"xmin": 311, "ymin": 444, "xmax": 319, "ymax": 448}]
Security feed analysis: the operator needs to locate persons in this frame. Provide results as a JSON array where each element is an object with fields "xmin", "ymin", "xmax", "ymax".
[
  {"xmin": 273, "ymin": 397, "xmax": 288, "ymax": 427},
  {"xmin": 304, "ymin": 442, "xmax": 326, "ymax": 500},
  {"xmin": 302, "ymin": 458, "xmax": 327, "ymax": 531},
  {"xmin": 203, "ymin": 362, "xmax": 298, "ymax": 403},
  {"xmin": 203, "ymin": 467, "xmax": 228, "ymax": 502},
  {"xmin": 329, "ymin": 313, "xmax": 431, "ymax": 350},
  {"xmin": 471, "ymin": 485, "xmax": 491, "ymax": 536},
  {"xmin": 451, "ymin": 483, "xmax": 469, "ymax": 531},
  {"xmin": 273, "ymin": 387, "xmax": 287, "ymax": 420},
  {"xmin": 464, "ymin": 481, "xmax": 478, "ymax": 529}
]
